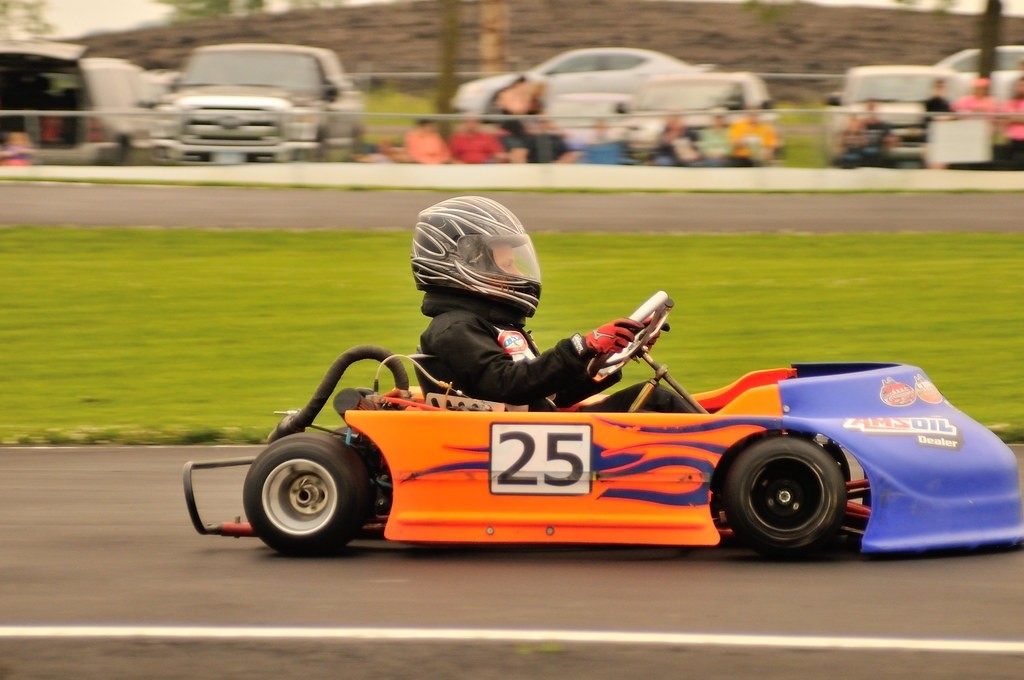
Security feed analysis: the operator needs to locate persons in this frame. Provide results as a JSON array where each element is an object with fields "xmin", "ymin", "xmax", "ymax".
[
  {"xmin": 412, "ymin": 197, "xmax": 697, "ymax": 416},
  {"xmin": 403, "ymin": 76, "xmax": 1024, "ymax": 166}
]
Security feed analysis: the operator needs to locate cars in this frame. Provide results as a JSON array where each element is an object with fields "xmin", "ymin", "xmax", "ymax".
[
  {"xmin": 451, "ymin": 46, "xmax": 773, "ymax": 115},
  {"xmin": 824, "ymin": 44, "xmax": 1024, "ymax": 160},
  {"xmin": 2, "ymin": 39, "xmax": 364, "ymax": 162}
]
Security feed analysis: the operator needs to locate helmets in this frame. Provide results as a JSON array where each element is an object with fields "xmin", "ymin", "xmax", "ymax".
[{"xmin": 411, "ymin": 195, "xmax": 543, "ymax": 315}]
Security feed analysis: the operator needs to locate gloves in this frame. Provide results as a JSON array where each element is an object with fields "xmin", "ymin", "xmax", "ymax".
[
  {"xmin": 643, "ymin": 315, "xmax": 671, "ymax": 351},
  {"xmin": 571, "ymin": 318, "xmax": 646, "ymax": 356}
]
